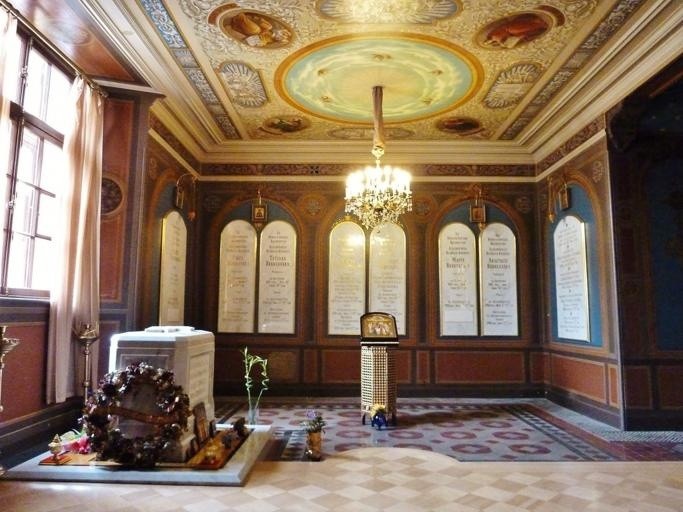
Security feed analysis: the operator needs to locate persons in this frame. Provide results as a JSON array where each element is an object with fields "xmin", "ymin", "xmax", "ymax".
[
  {"xmin": 230, "ymin": 11, "xmax": 291, "ymax": 48},
  {"xmin": 482, "ymin": 15, "xmax": 548, "ymax": 50}
]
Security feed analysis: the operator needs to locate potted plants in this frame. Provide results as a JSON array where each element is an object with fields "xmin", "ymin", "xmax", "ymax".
[{"xmin": 237, "ymin": 344, "xmax": 271, "ymax": 426}]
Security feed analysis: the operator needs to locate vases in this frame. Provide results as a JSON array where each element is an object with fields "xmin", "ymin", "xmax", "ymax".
[{"xmin": 308, "ymin": 434, "xmax": 322, "ymax": 461}]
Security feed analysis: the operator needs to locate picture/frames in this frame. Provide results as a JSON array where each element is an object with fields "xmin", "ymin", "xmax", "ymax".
[
  {"xmin": 251, "ymin": 203, "xmax": 267, "ymax": 222},
  {"xmin": 470, "ymin": 203, "xmax": 486, "ymax": 223}
]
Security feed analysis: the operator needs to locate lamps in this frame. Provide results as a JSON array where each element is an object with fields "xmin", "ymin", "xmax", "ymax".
[{"xmin": 344, "ymin": 84, "xmax": 414, "ymax": 230}]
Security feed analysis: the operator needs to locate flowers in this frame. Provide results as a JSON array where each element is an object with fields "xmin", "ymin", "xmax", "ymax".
[
  {"xmin": 79, "ymin": 361, "xmax": 191, "ymax": 469},
  {"xmin": 301, "ymin": 407, "xmax": 327, "ymax": 434}
]
